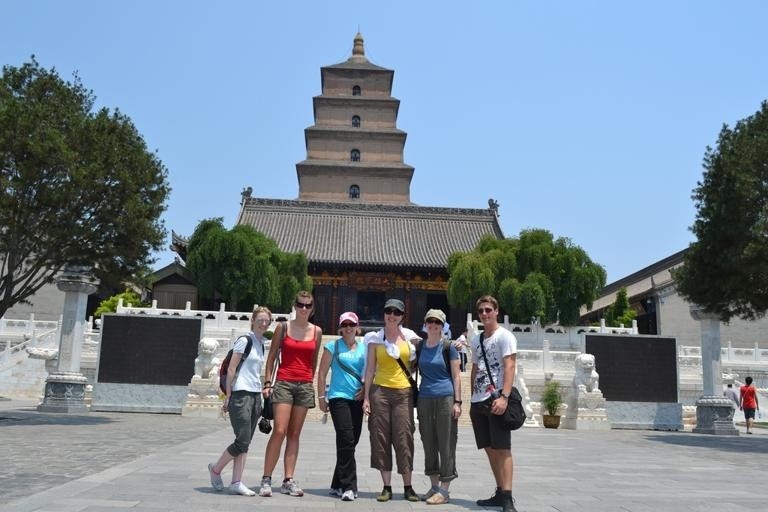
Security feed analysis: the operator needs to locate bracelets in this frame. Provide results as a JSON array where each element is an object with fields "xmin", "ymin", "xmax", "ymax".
[
  {"xmin": 318, "ymin": 395, "xmax": 325, "ymax": 399},
  {"xmin": 454, "ymin": 400, "xmax": 462, "ymax": 405},
  {"xmin": 264, "ymin": 380, "xmax": 271, "ymax": 385}
]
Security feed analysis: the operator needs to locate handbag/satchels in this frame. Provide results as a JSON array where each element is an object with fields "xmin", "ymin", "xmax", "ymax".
[
  {"xmin": 263, "ymin": 392, "xmax": 273, "ymax": 419},
  {"xmin": 411, "ymin": 379, "xmax": 418, "ymax": 407},
  {"xmin": 491, "ymin": 386, "xmax": 526, "ymax": 430}
]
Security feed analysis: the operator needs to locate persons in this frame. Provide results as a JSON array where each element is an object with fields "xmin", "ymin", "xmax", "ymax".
[
  {"xmin": 530, "ymin": 316, "xmax": 537, "ymax": 332},
  {"xmin": 207, "ymin": 305, "xmax": 274, "ymax": 496},
  {"xmin": 469, "ymin": 295, "xmax": 519, "ymax": 511},
  {"xmin": 259, "ymin": 291, "xmax": 322, "ymax": 496},
  {"xmin": 361, "ymin": 298, "xmax": 462, "ymax": 502},
  {"xmin": 739, "ymin": 376, "xmax": 759, "ymax": 434},
  {"xmin": 723, "ymin": 382, "xmax": 739, "ymax": 418},
  {"xmin": 455, "ymin": 327, "xmax": 470, "ymax": 373},
  {"xmin": 411, "ymin": 308, "xmax": 462, "ymax": 506},
  {"xmin": 316, "ymin": 311, "xmax": 368, "ymax": 500}
]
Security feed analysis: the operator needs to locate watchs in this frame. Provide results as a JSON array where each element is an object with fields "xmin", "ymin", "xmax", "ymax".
[{"xmin": 499, "ymin": 393, "xmax": 510, "ymax": 399}]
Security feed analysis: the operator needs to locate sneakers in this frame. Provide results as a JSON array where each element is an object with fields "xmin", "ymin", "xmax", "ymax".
[
  {"xmin": 342, "ymin": 489, "xmax": 358, "ymax": 501},
  {"xmin": 208, "ymin": 463, "xmax": 224, "ymax": 491},
  {"xmin": 427, "ymin": 492, "xmax": 449, "ymax": 504},
  {"xmin": 281, "ymin": 479, "xmax": 303, "ymax": 496},
  {"xmin": 228, "ymin": 481, "xmax": 255, "ymax": 496},
  {"xmin": 329, "ymin": 488, "xmax": 343, "ymax": 497},
  {"xmin": 377, "ymin": 489, "xmax": 392, "ymax": 500},
  {"xmin": 421, "ymin": 489, "xmax": 436, "ymax": 500},
  {"xmin": 259, "ymin": 478, "xmax": 272, "ymax": 496},
  {"xmin": 404, "ymin": 487, "xmax": 418, "ymax": 501}
]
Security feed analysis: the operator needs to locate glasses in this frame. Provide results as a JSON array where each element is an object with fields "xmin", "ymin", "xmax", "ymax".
[
  {"xmin": 341, "ymin": 323, "xmax": 356, "ymax": 327},
  {"xmin": 427, "ymin": 318, "xmax": 442, "ymax": 324},
  {"xmin": 478, "ymin": 308, "xmax": 492, "ymax": 312},
  {"xmin": 385, "ymin": 308, "xmax": 401, "ymax": 316},
  {"xmin": 296, "ymin": 302, "xmax": 311, "ymax": 308}
]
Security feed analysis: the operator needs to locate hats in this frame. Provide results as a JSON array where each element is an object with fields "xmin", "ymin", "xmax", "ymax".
[
  {"xmin": 424, "ymin": 309, "xmax": 446, "ymax": 325},
  {"xmin": 339, "ymin": 312, "xmax": 359, "ymax": 325},
  {"xmin": 385, "ymin": 299, "xmax": 405, "ymax": 312}
]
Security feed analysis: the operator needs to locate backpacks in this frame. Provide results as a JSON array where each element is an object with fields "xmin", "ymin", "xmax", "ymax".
[{"xmin": 219, "ymin": 335, "xmax": 252, "ymax": 394}]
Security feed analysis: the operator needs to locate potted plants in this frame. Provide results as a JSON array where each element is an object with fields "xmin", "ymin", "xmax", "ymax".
[{"xmin": 539, "ymin": 381, "xmax": 562, "ymax": 428}]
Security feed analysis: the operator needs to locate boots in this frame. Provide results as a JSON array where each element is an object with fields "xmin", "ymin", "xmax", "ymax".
[
  {"xmin": 501, "ymin": 490, "xmax": 517, "ymax": 512},
  {"xmin": 477, "ymin": 486, "xmax": 504, "ymax": 506}
]
